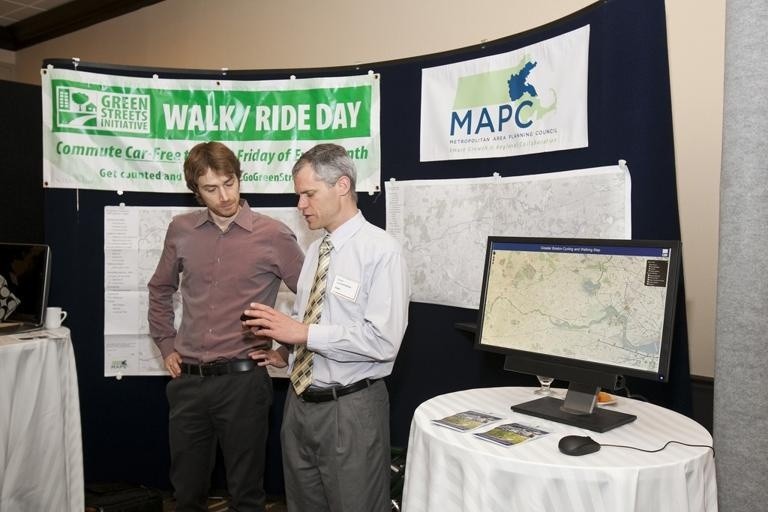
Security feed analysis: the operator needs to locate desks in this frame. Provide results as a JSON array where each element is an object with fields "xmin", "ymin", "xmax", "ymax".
[
  {"xmin": 1, "ymin": 326, "xmax": 76, "ymax": 510},
  {"xmin": 399, "ymin": 382, "xmax": 723, "ymax": 511}
]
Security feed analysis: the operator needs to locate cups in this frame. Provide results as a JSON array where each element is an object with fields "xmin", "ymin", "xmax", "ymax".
[{"xmin": 44, "ymin": 307, "xmax": 67, "ymax": 330}]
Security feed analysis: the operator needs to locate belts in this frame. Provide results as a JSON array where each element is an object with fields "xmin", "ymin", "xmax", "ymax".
[
  {"xmin": 180, "ymin": 361, "xmax": 253, "ymax": 375},
  {"xmin": 302, "ymin": 380, "xmax": 376, "ymax": 402}
]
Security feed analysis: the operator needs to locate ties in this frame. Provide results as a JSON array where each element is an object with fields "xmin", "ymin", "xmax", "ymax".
[{"xmin": 290, "ymin": 235, "xmax": 334, "ymax": 397}]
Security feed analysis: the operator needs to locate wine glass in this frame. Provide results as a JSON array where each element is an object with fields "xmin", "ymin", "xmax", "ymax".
[{"xmin": 533, "ymin": 375, "xmax": 557, "ymax": 396}]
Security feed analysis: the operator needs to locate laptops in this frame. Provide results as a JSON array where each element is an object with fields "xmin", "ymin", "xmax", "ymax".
[{"xmin": 0, "ymin": 242, "xmax": 53, "ymax": 336}]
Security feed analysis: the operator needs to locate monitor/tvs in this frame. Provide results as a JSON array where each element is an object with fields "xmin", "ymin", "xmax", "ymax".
[{"xmin": 472, "ymin": 235, "xmax": 683, "ymax": 433}]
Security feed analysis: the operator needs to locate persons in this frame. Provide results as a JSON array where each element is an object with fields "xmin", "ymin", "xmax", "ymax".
[
  {"xmin": 147, "ymin": 141, "xmax": 308, "ymax": 510},
  {"xmin": 241, "ymin": 142, "xmax": 409, "ymax": 511}
]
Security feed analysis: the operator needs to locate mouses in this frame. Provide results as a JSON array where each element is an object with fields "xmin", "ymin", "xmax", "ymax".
[{"xmin": 558, "ymin": 434, "xmax": 600, "ymax": 456}]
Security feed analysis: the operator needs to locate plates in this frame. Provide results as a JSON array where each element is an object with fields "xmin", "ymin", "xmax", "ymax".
[{"xmin": 597, "ymin": 396, "xmax": 618, "ymax": 406}]
